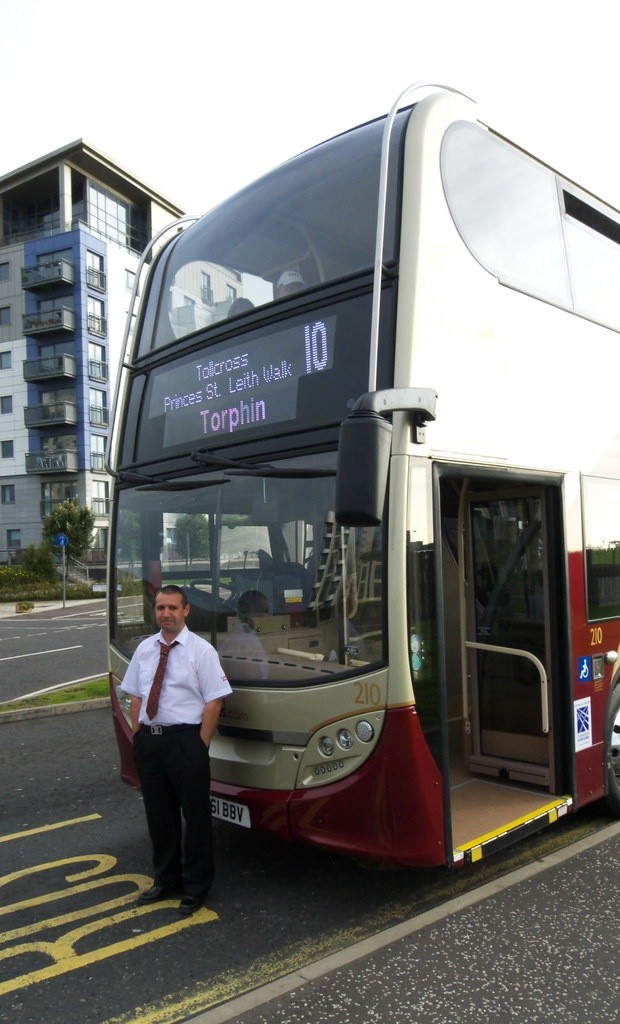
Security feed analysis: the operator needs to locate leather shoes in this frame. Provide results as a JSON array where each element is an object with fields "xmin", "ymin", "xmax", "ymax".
[
  {"xmin": 137, "ymin": 882, "xmax": 180, "ymax": 904},
  {"xmin": 179, "ymin": 889, "xmax": 209, "ymax": 912}
]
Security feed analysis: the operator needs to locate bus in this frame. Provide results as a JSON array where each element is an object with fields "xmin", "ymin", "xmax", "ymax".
[{"xmin": 98, "ymin": 76, "xmax": 620, "ymax": 879}]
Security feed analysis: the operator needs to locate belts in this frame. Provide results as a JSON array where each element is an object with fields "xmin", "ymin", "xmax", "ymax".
[{"xmin": 140, "ymin": 724, "xmax": 201, "ymax": 734}]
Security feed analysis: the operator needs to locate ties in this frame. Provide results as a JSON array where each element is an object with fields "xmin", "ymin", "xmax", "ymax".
[{"xmin": 146, "ymin": 643, "xmax": 178, "ymax": 721}]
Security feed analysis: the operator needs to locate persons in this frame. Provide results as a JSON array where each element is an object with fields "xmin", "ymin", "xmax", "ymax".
[
  {"xmin": 116, "ymin": 585, "xmax": 233, "ymax": 909},
  {"xmin": 210, "ymin": 589, "xmax": 270, "ymax": 687}
]
